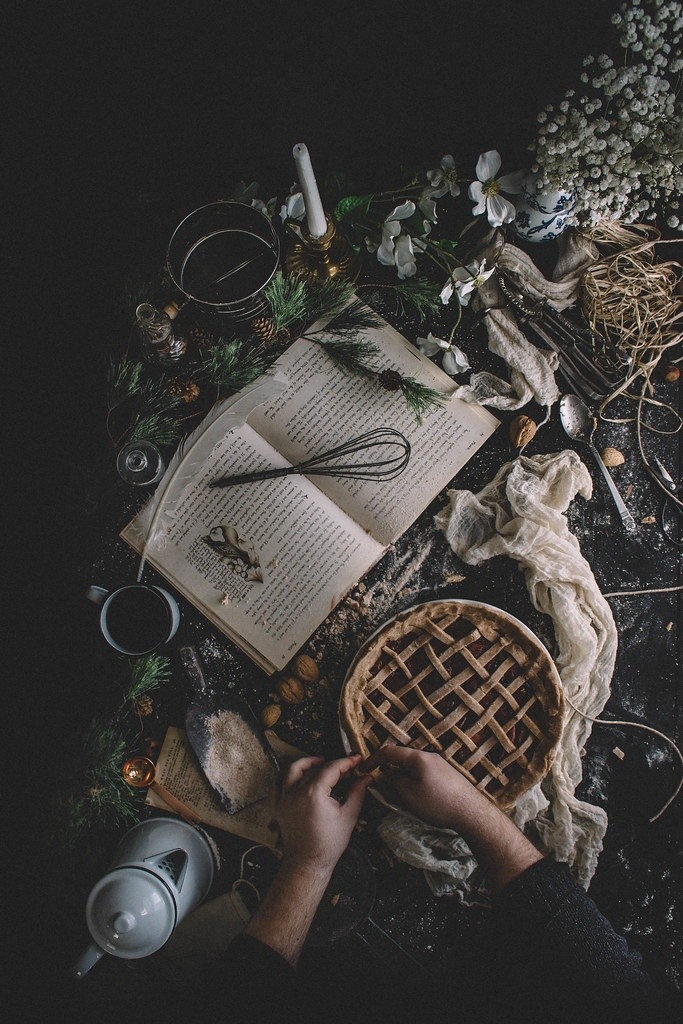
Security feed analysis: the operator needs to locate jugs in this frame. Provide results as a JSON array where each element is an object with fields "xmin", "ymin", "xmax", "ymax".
[{"xmin": 48, "ymin": 817, "xmax": 219, "ymax": 991}]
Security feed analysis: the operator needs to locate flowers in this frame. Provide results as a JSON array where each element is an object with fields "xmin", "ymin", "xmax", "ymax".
[
  {"xmin": 526, "ymin": 1, "xmax": 683, "ymax": 234},
  {"xmin": 206, "ymin": 147, "xmax": 529, "ymax": 377}
]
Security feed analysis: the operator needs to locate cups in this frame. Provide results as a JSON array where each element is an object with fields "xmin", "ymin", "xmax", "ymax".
[
  {"xmin": 83, "ymin": 582, "xmax": 181, "ymax": 658},
  {"xmin": 513, "ymin": 170, "xmax": 579, "ymax": 245}
]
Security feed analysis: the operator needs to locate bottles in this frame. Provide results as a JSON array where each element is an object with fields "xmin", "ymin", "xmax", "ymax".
[
  {"xmin": 133, "ymin": 304, "xmax": 176, "ymax": 356},
  {"xmin": 116, "ymin": 440, "xmax": 166, "ymax": 489}
]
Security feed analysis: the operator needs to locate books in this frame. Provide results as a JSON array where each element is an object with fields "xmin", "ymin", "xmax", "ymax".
[{"xmin": 119, "ymin": 294, "xmax": 501, "ymax": 677}]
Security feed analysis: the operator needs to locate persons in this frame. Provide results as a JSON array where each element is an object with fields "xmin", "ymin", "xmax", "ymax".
[{"xmin": 186, "ymin": 746, "xmax": 657, "ymax": 1024}]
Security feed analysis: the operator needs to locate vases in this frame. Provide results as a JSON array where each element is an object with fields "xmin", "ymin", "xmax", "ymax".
[{"xmin": 509, "ymin": 156, "xmax": 580, "ymax": 244}]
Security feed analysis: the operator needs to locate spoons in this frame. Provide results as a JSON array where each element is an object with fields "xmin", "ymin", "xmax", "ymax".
[
  {"xmin": 119, "ymin": 757, "xmax": 202, "ymax": 826},
  {"xmin": 560, "ymin": 394, "xmax": 637, "ymax": 532}
]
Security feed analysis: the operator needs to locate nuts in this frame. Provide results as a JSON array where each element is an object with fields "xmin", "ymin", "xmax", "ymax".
[
  {"xmin": 510, "ymin": 416, "xmax": 536, "ymax": 448},
  {"xmin": 295, "ymin": 655, "xmax": 318, "ymax": 681},
  {"xmin": 278, "ymin": 675, "xmax": 304, "ymax": 703},
  {"xmin": 261, "ymin": 703, "xmax": 281, "ymax": 726},
  {"xmin": 602, "ymin": 448, "xmax": 624, "ymax": 466}
]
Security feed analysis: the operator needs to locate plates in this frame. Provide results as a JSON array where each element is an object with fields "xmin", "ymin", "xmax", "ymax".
[{"xmin": 336, "ymin": 598, "xmax": 564, "ymax": 829}]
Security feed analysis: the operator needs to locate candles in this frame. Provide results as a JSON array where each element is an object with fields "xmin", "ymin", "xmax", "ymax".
[{"xmin": 292, "ymin": 143, "xmax": 328, "ymax": 240}]
